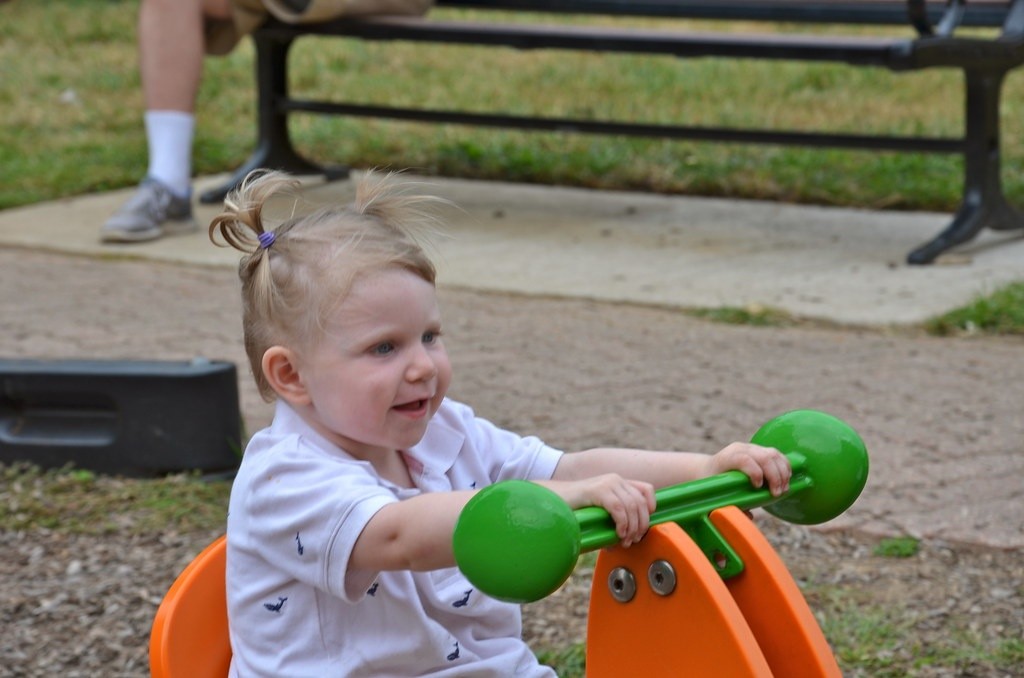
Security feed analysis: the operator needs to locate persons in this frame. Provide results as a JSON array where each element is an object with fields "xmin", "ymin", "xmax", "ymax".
[
  {"xmin": 209, "ymin": 168, "xmax": 792, "ymax": 678},
  {"xmin": 103, "ymin": 0, "xmax": 430, "ymax": 241}
]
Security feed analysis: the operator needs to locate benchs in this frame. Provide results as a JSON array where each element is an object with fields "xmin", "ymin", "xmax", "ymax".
[{"xmin": 201, "ymin": 0, "xmax": 1024, "ymax": 267}]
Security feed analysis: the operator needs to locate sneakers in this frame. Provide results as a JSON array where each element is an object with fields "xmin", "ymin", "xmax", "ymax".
[{"xmin": 101, "ymin": 174, "xmax": 195, "ymax": 241}]
